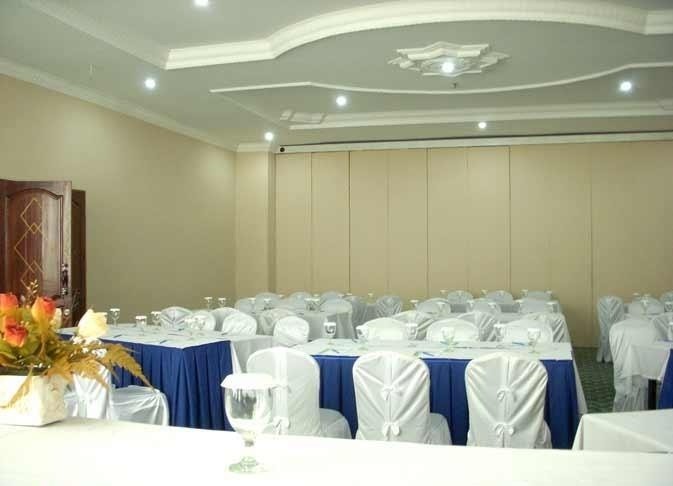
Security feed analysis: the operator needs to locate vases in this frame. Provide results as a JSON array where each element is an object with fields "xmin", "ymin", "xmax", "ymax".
[{"xmin": 1, "ymin": 371, "xmax": 73, "ymax": 430}]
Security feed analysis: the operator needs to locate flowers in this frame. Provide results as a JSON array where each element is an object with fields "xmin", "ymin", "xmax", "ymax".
[{"xmin": 0, "ymin": 278, "xmax": 157, "ymax": 413}]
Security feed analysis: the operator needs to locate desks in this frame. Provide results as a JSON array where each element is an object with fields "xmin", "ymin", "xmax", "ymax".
[{"xmin": 1, "ymin": 416, "xmax": 673, "ymax": 486}]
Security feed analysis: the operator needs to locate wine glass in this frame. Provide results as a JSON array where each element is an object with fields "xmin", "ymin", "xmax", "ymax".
[
  {"xmin": 223, "ymin": 382, "xmax": 273, "ymax": 472},
  {"xmin": 108, "ymin": 308, "xmax": 119, "ymax": 329},
  {"xmin": 304, "ymin": 291, "xmax": 374, "ymax": 312},
  {"xmin": 151, "ymin": 312, "xmax": 160, "ymax": 332},
  {"xmin": 403, "ymin": 322, "xmax": 417, "ymax": 347},
  {"xmin": 441, "ymin": 327, "xmax": 456, "ymax": 353},
  {"xmin": 494, "ymin": 324, "xmax": 506, "ymax": 348},
  {"xmin": 135, "ymin": 315, "xmax": 146, "ymax": 335},
  {"xmin": 356, "ymin": 325, "xmax": 369, "ymax": 350},
  {"xmin": 203, "ymin": 292, "xmax": 287, "ymax": 311},
  {"xmin": 323, "ymin": 322, "xmax": 336, "ymax": 344},
  {"xmin": 632, "ymin": 291, "xmax": 672, "ymax": 341},
  {"xmin": 409, "ymin": 288, "xmax": 555, "ymax": 321},
  {"xmin": 527, "ymin": 327, "xmax": 539, "ymax": 355}
]
